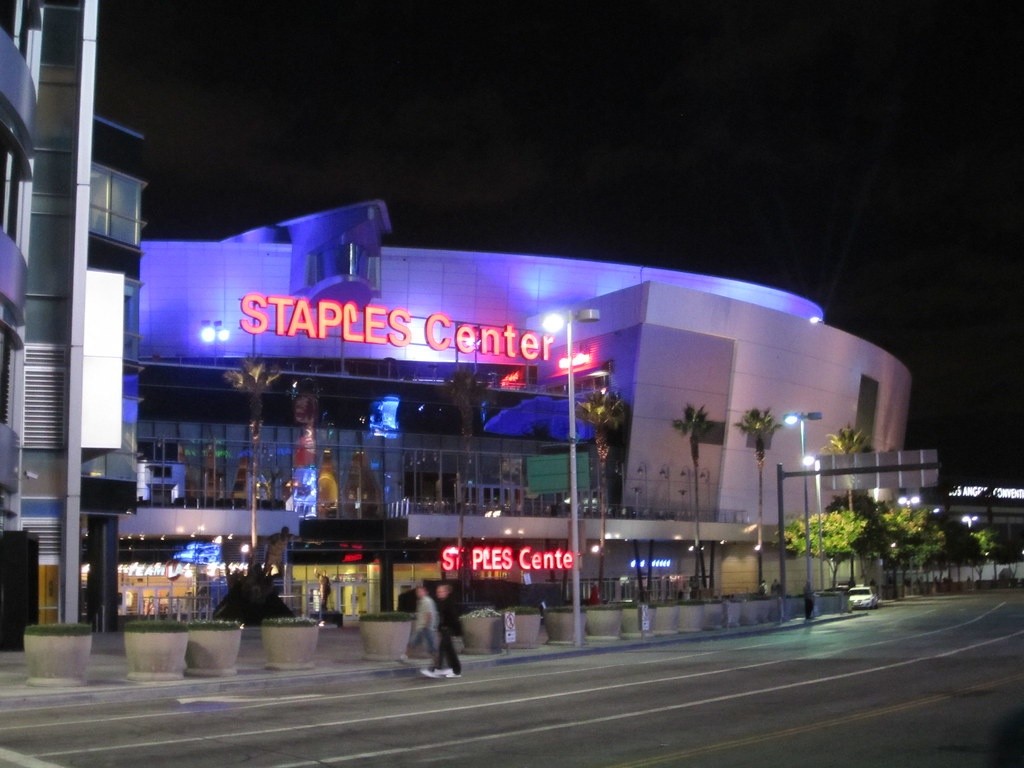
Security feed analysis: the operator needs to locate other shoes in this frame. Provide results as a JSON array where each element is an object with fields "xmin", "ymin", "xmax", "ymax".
[
  {"xmin": 446, "ymin": 672, "xmax": 463, "ymax": 680},
  {"xmin": 420, "ymin": 669, "xmax": 441, "ymax": 679}
]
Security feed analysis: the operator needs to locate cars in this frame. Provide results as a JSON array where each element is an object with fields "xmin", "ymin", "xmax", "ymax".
[{"xmin": 847, "ymin": 586, "xmax": 879, "ymax": 610}]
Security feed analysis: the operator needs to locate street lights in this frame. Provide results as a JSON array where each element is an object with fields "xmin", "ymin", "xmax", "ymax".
[
  {"xmin": 541, "ymin": 307, "xmax": 600, "ymax": 651},
  {"xmin": 783, "ymin": 411, "xmax": 824, "ymax": 619}
]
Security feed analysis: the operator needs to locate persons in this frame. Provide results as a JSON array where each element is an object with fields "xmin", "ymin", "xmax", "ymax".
[
  {"xmin": 400, "ymin": 586, "xmax": 438, "ymax": 663},
  {"xmin": 760, "ymin": 580, "xmax": 767, "ymax": 596},
  {"xmin": 315, "ymin": 566, "xmax": 331, "ymax": 610},
  {"xmin": 804, "ymin": 582, "xmax": 815, "ymax": 619},
  {"xmin": 420, "ymin": 584, "xmax": 463, "ymax": 678},
  {"xmin": 771, "ymin": 580, "xmax": 779, "ymax": 593}
]
